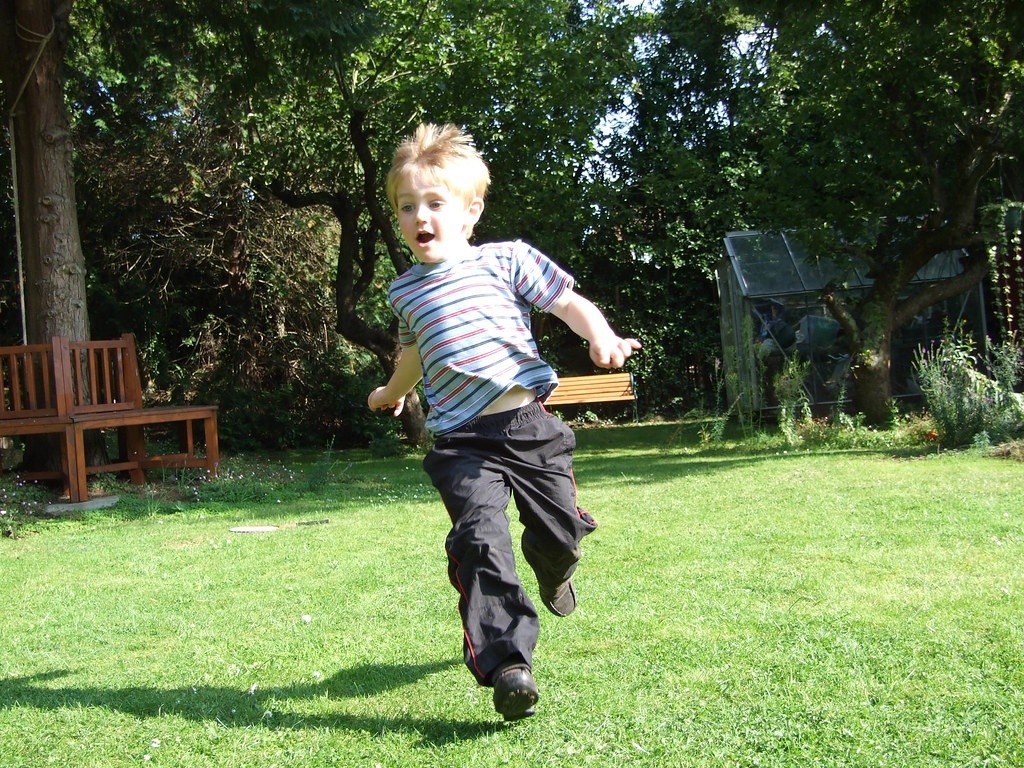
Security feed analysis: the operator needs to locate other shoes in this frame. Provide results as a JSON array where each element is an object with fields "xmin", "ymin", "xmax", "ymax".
[
  {"xmin": 493, "ymin": 668, "xmax": 538, "ymax": 721},
  {"xmin": 521, "ymin": 529, "xmax": 576, "ymax": 617}
]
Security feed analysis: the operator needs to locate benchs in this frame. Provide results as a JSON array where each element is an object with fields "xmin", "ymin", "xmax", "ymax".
[
  {"xmin": 0, "ymin": 333, "xmax": 220, "ymax": 504},
  {"xmin": 543, "ymin": 371, "xmax": 639, "ymax": 425}
]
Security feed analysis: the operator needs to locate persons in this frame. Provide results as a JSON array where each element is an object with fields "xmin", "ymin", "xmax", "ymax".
[
  {"xmin": 368, "ymin": 122, "xmax": 642, "ymax": 720},
  {"xmin": 757, "ymin": 304, "xmax": 783, "ymax": 338}
]
